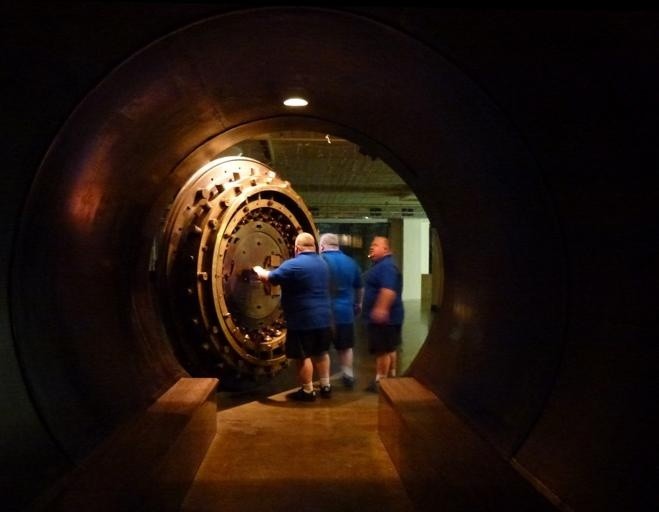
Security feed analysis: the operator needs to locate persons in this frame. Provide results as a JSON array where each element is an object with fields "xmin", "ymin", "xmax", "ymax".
[
  {"xmin": 318, "ymin": 233, "xmax": 366, "ymax": 390},
  {"xmin": 252, "ymin": 231, "xmax": 337, "ymax": 403},
  {"xmin": 359, "ymin": 233, "xmax": 407, "ymax": 394}
]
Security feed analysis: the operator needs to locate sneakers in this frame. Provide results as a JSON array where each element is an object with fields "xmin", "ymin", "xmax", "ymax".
[
  {"xmin": 320, "ymin": 383, "xmax": 334, "ymax": 398},
  {"xmin": 286, "ymin": 387, "xmax": 318, "ymax": 402},
  {"xmin": 362, "ymin": 381, "xmax": 385, "ymax": 393},
  {"xmin": 339, "ymin": 372, "xmax": 355, "ymax": 390}
]
